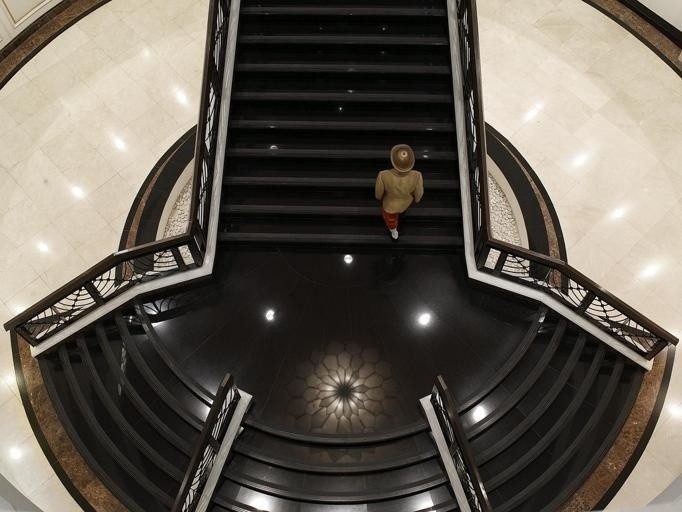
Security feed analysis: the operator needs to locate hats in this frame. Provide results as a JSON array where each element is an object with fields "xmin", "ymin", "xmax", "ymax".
[{"xmin": 390, "ymin": 144, "xmax": 415, "ymax": 172}]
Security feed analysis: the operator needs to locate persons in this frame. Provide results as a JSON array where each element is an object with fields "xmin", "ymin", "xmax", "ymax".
[{"xmin": 374, "ymin": 144, "xmax": 424, "ymax": 241}]
